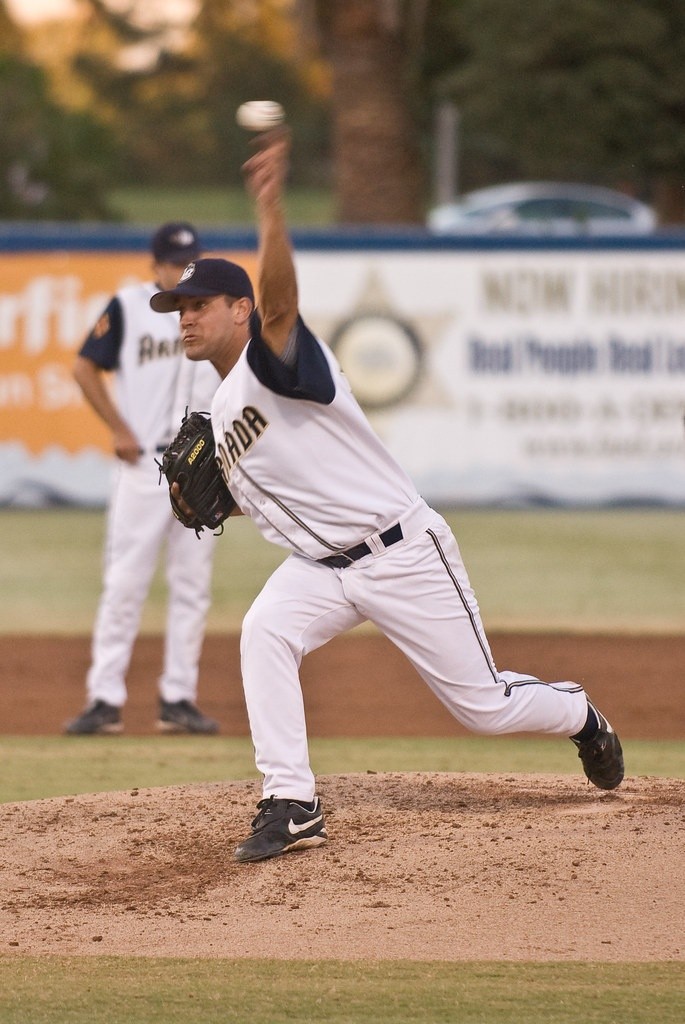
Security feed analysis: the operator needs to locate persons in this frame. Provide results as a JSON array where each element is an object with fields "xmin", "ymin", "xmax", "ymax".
[
  {"xmin": 72, "ymin": 221, "xmax": 222, "ymax": 738},
  {"xmin": 149, "ymin": 125, "xmax": 624, "ymax": 860}
]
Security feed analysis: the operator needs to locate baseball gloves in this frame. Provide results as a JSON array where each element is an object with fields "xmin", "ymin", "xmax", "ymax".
[{"xmin": 152, "ymin": 404, "xmax": 238, "ymax": 540}]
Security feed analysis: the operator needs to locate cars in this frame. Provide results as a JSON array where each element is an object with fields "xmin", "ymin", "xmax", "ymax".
[{"xmin": 428, "ymin": 181, "xmax": 660, "ymax": 238}]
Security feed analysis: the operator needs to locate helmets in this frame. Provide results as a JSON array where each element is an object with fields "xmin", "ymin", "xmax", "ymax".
[{"xmin": 151, "ymin": 221, "xmax": 200, "ymax": 263}]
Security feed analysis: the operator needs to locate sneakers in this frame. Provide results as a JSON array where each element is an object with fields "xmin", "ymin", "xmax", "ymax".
[
  {"xmin": 155, "ymin": 696, "xmax": 217, "ymax": 734},
  {"xmin": 235, "ymin": 794, "xmax": 328, "ymax": 863},
  {"xmin": 569, "ymin": 692, "xmax": 624, "ymax": 790},
  {"xmin": 66, "ymin": 700, "xmax": 124, "ymax": 732}
]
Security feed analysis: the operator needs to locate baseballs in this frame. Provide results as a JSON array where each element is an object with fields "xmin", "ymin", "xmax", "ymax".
[{"xmin": 237, "ymin": 100, "xmax": 285, "ymax": 130}]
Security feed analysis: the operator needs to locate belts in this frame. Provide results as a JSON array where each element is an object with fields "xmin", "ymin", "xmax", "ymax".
[
  {"xmin": 316, "ymin": 523, "xmax": 403, "ymax": 569},
  {"xmin": 139, "ymin": 446, "xmax": 168, "ymax": 455}
]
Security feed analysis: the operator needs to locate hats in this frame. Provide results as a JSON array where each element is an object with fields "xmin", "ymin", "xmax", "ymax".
[{"xmin": 150, "ymin": 259, "xmax": 255, "ymax": 312}]
